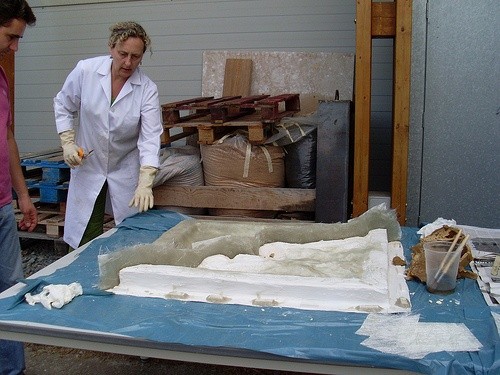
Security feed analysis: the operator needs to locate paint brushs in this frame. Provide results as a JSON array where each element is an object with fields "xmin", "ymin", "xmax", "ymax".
[
  {"xmin": 429, "ymin": 234, "xmax": 470, "ymax": 293},
  {"xmin": 428, "ymin": 229, "xmax": 464, "ymax": 290}
]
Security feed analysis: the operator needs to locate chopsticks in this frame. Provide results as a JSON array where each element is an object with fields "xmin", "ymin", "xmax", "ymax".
[{"xmin": 434, "ymin": 229, "xmax": 470, "ymax": 284}]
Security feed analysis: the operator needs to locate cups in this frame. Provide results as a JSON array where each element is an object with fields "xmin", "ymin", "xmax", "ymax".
[{"xmin": 423, "ymin": 241, "xmax": 464, "ymax": 294}]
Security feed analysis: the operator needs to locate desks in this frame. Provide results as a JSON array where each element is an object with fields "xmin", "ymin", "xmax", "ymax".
[{"xmin": 0, "ymin": 214, "xmax": 500, "ymax": 375}]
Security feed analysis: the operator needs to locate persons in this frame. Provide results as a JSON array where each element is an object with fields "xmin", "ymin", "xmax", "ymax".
[
  {"xmin": 53, "ymin": 22, "xmax": 163, "ymax": 255},
  {"xmin": 0, "ymin": 0, "xmax": 37, "ymax": 375}
]
